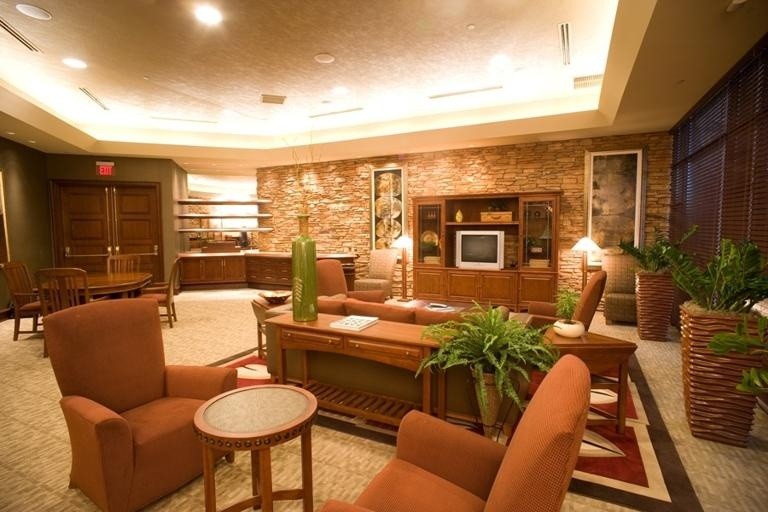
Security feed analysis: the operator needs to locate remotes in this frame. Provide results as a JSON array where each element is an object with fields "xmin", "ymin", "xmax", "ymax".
[{"xmin": 431, "ymin": 304, "xmax": 448, "ymax": 307}]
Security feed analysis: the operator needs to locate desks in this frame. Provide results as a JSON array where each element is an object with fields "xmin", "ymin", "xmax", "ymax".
[
  {"xmin": 386, "ymin": 297, "xmax": 491, "ymax": 313},
  {"xmin": 265, "ymin": 311, "xmax": 460, "ymax": 443},
  {"xmin": 534, "ymin": 320, "xmax": 637, "ymax": 438},
  {"xmin": 252, "ymin": 298, "xmax": 296, "ymax": 360},
  {"xmin": 191, "ymin": 383, "xmax": 316, "ymax": 511}
]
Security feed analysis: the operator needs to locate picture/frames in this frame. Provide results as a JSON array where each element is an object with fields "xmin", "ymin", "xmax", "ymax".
[
  {"xmin": 370, "ymin": 166, "xmax": 406, "ymax": 262},
  {"xmin": 585, "ymin": 147, "xmax": 640, "ymax": 268}
]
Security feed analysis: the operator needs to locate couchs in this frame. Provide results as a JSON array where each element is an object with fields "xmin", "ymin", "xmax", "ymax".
[{"xmin": 316, "ymin": 296, "xmax": 511, "ymax": 331}]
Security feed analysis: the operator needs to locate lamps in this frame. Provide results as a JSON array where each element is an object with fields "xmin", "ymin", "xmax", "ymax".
[
  {"xmin": 570, "ymin": 234, "xmax": 602, "ymax": 294},
  {"xmin": 390, "ymin": 235, "xmax": 413, "ymax": 301}
]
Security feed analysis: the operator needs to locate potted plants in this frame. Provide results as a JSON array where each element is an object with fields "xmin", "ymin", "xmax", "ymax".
[
  {"xmin": 414, "ymin": 300, "xmax": 562, "ymax": 441},
  {"xmin": 663, "ymin": 232, "xmax": 766, "ymax": 451},
  {"xmin": 552, "ymin": 287, "xmax": 586, "ymax": 340},
  {"xmin": 618, "ymin": 226, "xmax": 705, "ymax": 343}
]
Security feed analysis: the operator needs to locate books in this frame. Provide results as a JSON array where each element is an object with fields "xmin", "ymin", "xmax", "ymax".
[{"xmin": 330, "ymin": 315, "xmax": 379, "ymax": 331}]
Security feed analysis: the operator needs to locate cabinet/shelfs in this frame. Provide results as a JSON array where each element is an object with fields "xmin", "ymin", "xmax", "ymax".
[
  {"xmin": 318, "ymin": 258, "xmax": 357, "ymax": 291},
  {"xmin": 247, "ymin": 255, "xmax": 291, "ymax": 285},
  {"xmin": 446, "ymin": 269, "xmax": 518, "ymax": 314},
  {"xmin": 176, "ymin": 197, "xmax": 275, "ymax": 235},
  {"xmin": 413, "ymin": 267, "xmax": 447, "ymax": 301},
  {"xmin": 412, "ymin": 195, "xmax": 455, "ymax": 268},
  {"xmin": 204, "ymin": 256, "xmax": 247, "ymax": 282},
  {"xmin": 518, "ymin": 272, "xmax": 558, "ymax": 314},
  {"xmin": 519, "ymin": 188, "xmax": 562, "ymax": 274},
  {"xmin": 444, "ymin": 191, "xmax": 520, "ymax": 225},
  {"xmin": 177, "ymin": 256, "xmax": 204, "ymax": 285}
]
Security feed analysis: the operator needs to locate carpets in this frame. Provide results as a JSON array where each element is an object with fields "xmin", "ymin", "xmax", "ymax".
[{"xmin": 200, "ymin": 340, "xmax": 701, "ymax": 511}]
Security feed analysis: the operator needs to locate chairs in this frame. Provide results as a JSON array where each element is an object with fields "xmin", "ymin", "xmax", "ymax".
[
  {"xmin": 314, "ymin": 346, "xmax": 595, "ymax": 511},
  {"xmin": 0, "ymin": 250, "xmax": 185, "ymax": 358},
  {"xmin": 312, "ymin": 256, "xmax": 384, "ymax": 304},
  {"xmin": 603, "ymin": 252, "xmax": 648, "ymax": 326},
  {"xmin": 41, "ymin": 295, "xmax": 239, "ymax": 511},
  {"xmin": 353, "ymin": 248, "xmax": 398, "ymax": 300},
  {"xmin": 524, "ymin": 271, "xmax": 607, "ymax": 333}
]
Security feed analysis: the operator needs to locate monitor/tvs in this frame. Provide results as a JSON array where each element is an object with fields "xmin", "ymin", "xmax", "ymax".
[{"xmin": 456, "ymin": 231, "xmax": 504, "ymax": 271}]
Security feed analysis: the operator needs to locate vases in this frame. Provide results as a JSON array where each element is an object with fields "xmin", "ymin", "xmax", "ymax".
[{"xmin": 291, "ymin": 212, "xmax": 316, "ymax": 324}]
